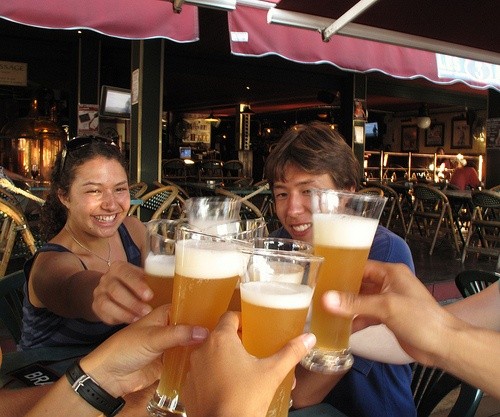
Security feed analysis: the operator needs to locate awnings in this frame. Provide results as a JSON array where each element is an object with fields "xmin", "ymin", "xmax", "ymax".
[
  {"xmin": 0, "ymin": 0, "xmax": 236, "ymax": 42},
  {"xmin": 228, "ymin": 0, "xmax": 500, "ymax": 92}
]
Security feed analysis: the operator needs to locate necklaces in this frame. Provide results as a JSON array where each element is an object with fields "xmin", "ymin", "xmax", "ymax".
[{"xmin": 65, "ymin": 225, "xmax": 111, "ymax": 266}]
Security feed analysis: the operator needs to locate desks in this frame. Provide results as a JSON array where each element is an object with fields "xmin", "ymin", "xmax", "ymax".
[
  {"xmin": 222, "ymin": 187, "xmax": 271, "ymax": 196},
  {"xmin": 438, "ymin": 189, "xmax": 485, "ymax": 250}
]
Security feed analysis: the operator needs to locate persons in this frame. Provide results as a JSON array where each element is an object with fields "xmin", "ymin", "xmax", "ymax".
[
  {"xmin": 0, "ymin": 166, "xmax": 36, "ymax": 216},
  {"xmin": 0, "ymin": 303, "xmax": 315, "ymax": 417},
  {"xmin": 18, "ymin": 137, "xmax": 154, "ymax": 351},
  {"xmin": 451, "ymin": 159, "xmax": 483, "ymax": 190},
  {"xmin": 269, "ymin": 124, "xmax": 418, "ymax": 417},
  {"xmin": 431, "ymin": 147, "xmax": 450, "ymax": 168},
  {"xmin": 322, "ymin": 261, "xmax": 500, "ymax": 400}
]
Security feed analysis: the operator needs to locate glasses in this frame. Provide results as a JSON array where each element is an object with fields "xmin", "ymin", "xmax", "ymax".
[{"xmin": 59, "ymin": 136, "xmax": 120, "ymax": 174}]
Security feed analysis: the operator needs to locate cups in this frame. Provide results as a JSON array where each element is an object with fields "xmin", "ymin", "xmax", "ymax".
[
  {"xmin": 139, "ymin": 197, "xmax": 325, "ymax": 417},
  {"xmin": 298, "ymin": 189, "xmax": 389, "ymax": 374}
]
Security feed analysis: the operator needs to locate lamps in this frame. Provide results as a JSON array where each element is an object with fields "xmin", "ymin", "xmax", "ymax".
[
  {"xmin": 204, "ymin": 112, "xmax": 218, "ymax": 121},
  {"xmin": 240, "ymin": 106, "xmax": 255, "ymax": 114},
  {"xmin": 416, "ymin": 107, "xmax": 431, "ymax": 129}
]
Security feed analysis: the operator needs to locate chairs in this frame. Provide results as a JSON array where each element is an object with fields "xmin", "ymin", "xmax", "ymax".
[
  {"xmin": 409, "ymin": 361, "xmax": 484, "ymax": 417},
  {"xmin": 129, "ymin": 157, "xmax": 273, "ymax": 235},
  {"xmin": 364, "ymin": 178, "xmax": 500, "ymax": 269},
  {"xmin": 0, "ymin": 164, "xmax": 51, "ymax": 350},
  {"xmin": 455, "ymin": 268, "xmax": 499, "ymax": 298}
]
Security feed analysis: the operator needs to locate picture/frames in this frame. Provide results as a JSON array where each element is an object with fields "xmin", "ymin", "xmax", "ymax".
[
  {"xmin": 400, "ymin": 125, "xmax": 419, "ymax": 152},
  {"xmin": 451, "ymin": 118, "xmax": 472, "ymax": 149},
  {"xmin": 425, "ymin": 123, "xmax": 444, "ymax": 147}
]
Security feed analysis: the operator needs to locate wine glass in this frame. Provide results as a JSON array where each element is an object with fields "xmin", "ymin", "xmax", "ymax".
[{"xmin": 30, "ymin": 164, "xmax": 39, "ymax": 182}]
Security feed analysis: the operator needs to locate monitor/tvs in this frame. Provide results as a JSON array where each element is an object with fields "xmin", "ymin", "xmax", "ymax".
[
  {"xmin": 366, "ymin": 121, "xmax": 380, "ymax": 139},
  {"xmin": 99, "ymin": 86, "xmax": 132, "ymax": 118},
  {"xmin": 179, "ymin": 147, "xmax": 191, "ymax": 159}
]
